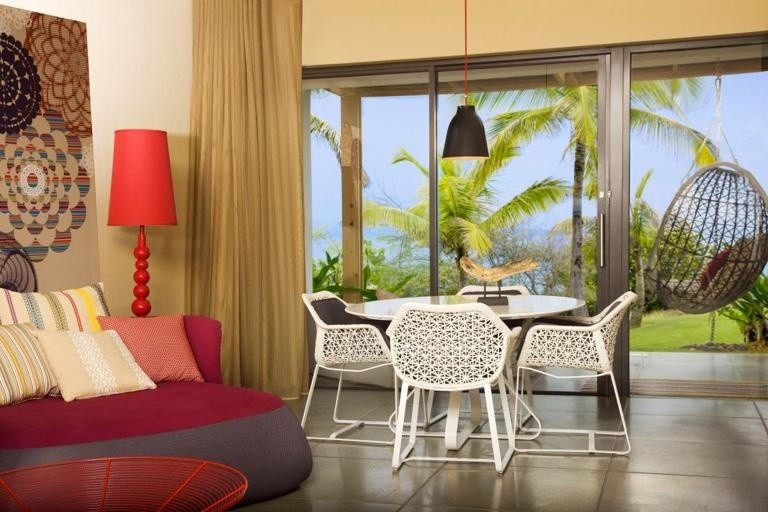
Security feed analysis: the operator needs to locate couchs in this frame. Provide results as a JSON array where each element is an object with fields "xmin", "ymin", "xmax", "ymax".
[{"xmin": 0, "ymin": 284, "xmax": 317, "ymax": 511}]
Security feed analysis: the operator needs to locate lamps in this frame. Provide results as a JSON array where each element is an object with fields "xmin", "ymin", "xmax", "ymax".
[
  {"xmin": 108, "ymin": 128, "xmax": 178, "ymax": 317},
  {"xmin": 443, "ymin": 0, "xmax": 488, "ymax": 159}
]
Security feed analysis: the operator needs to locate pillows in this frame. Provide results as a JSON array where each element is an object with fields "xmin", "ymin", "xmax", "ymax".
[
  {"xmin": 97, "ymin": 313, "xmax": 204, "ymax": 383},
  {"xmin": 0, "ymin": 322, "xmax": 53, "ymax": 405},
  {"xmin": 37, "ymin": 327, "xmax": 156, "ymax": 403},
  {"xmin": 0, "ymin": 283, "xmax": 108, "ymax": 330}
]
